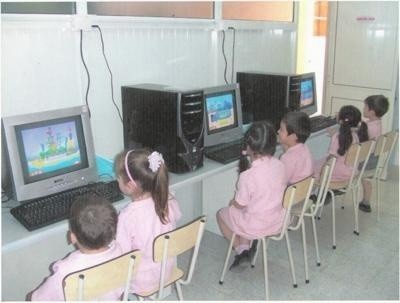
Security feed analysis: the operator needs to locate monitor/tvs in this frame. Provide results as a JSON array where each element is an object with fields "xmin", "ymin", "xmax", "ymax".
[
  {"xmin": 1, "ymin": 105, "xmax": 99, "ymax": 202},
  {"xmin": 296, "ymin": 73, "xmax": 318, "ymax": 119},
  {"xmin": 203, "ymin": 83, "xmax": 245, "ymax": 148}
]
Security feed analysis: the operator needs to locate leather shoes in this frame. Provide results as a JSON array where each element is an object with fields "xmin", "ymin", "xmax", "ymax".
[
  {"xmin": 229, "ymin": 250, "xmax": 251, "ymax": 273},
  {"xmin": 358, "ymin": 202, "xmax": 372, "ymax": 213}
]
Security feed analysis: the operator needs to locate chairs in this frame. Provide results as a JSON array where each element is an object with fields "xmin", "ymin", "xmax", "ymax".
[
  {"xmin": 342, "ymin": 129, "xmax": 398, "ymax": 219},
  {"xmin": 319, "ymin": 137, "xmax": 375, "ymax": 235},
  {"xmin": 61, "ymin": 249, "xmax": 141, "ymax": 303},
  {"xmin": 289, "ymin": 156, "xmax": 334, "ymax": 267},
  {"xmin": 219, "ymin": 174, "xmax": 315, "ymax": 297},
  {"xmin": 129, "ymin": 215, "xmax": 207, "ymax": 302}
]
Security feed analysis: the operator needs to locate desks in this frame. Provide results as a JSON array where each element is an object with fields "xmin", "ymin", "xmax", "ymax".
[{"xmin": 0, "ymin": 116, "xmax": 358, "ymax": 303}]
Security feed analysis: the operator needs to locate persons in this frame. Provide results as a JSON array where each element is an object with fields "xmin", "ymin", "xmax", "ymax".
[
  {"xmin": 278, "ymin": 111, "xmax": 314, "ymax": 227},
  {"xmin": 27, "ymin": 196, "xmax": 130, "ymax": 301},
  {"xmin": 313, "ymin": 104, "xmax": 368, "ymax": 196},
  {"xmin": 359, "ymin": 94, "xmax": 389, "ymax": 212},
  {"xmin": 217, "ymin": 121, "xmax": 286, "ymax": 272},
  {"xmin": 114, "ymin": 148, "xmax": 182, "ymax": 301}
]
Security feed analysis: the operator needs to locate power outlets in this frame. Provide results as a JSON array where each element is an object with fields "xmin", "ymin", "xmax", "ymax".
[
  {"xmin": 74, "ymin": 15, "xmax": 98, "ymax": 32},
  {"xmin": 220, "ymin": 21, "xmax": 237, "ymax": 30}
]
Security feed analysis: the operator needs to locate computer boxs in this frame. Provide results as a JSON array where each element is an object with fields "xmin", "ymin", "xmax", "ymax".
[
  {"xmin": 236, "ymin": 71, "xmax": 302, "ymax": 137},
  {"xmin": 122, "ymin": 83, "xmax": 206, "ymax": 175}
]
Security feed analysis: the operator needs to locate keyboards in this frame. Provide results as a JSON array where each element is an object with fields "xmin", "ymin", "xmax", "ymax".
[
  {"xmin": 11, "ymin": 182, "xmax": 125, "ymax": 230},
  {"xmin": 304, "ymin": 116, "xmax": 338, "ymax": 133},
  {"xmin": 204, "ymin": 142, "xmax": 250, "ymax": 165}
]
